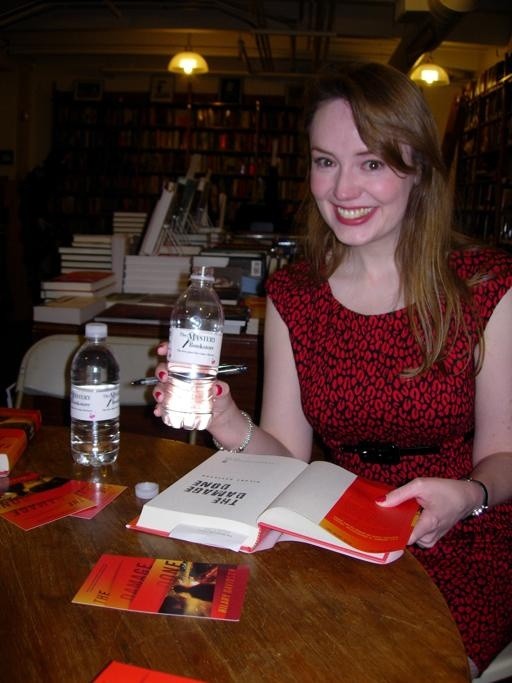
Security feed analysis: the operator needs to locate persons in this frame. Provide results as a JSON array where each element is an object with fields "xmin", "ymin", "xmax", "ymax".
[{"xmin": 149, "ymin": 61, "xmax": 511, "ymax": 680}]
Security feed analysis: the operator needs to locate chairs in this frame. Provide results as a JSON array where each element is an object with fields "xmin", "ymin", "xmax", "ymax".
[{"xmin": 12, "ymin": 332, "xmax": 199, "ymax": 445}]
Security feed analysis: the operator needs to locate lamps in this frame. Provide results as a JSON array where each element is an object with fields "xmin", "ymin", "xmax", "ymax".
[
  {"xmin": 406, "ymin": 46, "xmax": 453, "ymax": 88},
  {"xmin": 165, "ymin": 28, "xmax": 211, "ymax": 76}
]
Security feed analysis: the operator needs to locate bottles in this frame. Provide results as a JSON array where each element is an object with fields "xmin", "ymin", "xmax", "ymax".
[
  {"xmin": 160, "ymin": 264, "xmax": 225, "ymax": 431},
  {"xmin": 69, "ymin": 322, "xmax": 122, "ymax": 468}
]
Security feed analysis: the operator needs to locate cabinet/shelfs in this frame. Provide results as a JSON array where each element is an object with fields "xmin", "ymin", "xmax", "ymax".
[
  {"xmin": 37, "ymin": 65, "xmax": 313, "ymax": 271},
  {"xmin": 442, "ymin": 52, "xmax": 511, "ymax": 258}
]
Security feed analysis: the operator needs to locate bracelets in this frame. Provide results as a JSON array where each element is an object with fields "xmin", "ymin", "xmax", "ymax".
[
  {"xmin": 213, "ymin": 410, "xmax": 252, "ymax": 454},
  {"xmin": 464, "ymin": 476, "xmax": 489, "ymax": 517}
]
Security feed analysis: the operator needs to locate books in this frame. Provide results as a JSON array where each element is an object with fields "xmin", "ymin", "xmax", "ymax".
[
  {"xmin": 93, "ymin": 661, "xmax": 209, "ymax": 682},
  {"xmin": 125, "ymin": 450, "xmax": 422, "ymax": 564},
  {"xmin": 71, "ymin": 552, "xmax": 249, "ymax": 622},
  {"xmin": 1, "ymin": 407, "xmax": 43, "ymax": 477},
  {"xmin": 0, "ymin": 468, "xmax": 128, "ymax": 532}
]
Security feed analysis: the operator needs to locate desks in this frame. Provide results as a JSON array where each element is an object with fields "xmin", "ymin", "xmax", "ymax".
[
  {"xmin": 0, "ymin": 420, "xmax": 475, "ymax": 683},
  {"xmin": 10, "ymin": 293, "xmax": 263, "ymax": 453}
]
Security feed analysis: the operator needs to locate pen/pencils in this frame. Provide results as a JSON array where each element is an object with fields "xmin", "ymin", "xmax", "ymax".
[{"xmin": 129, "ymin": 362, "xmax": 248, "ymax": 387}]
